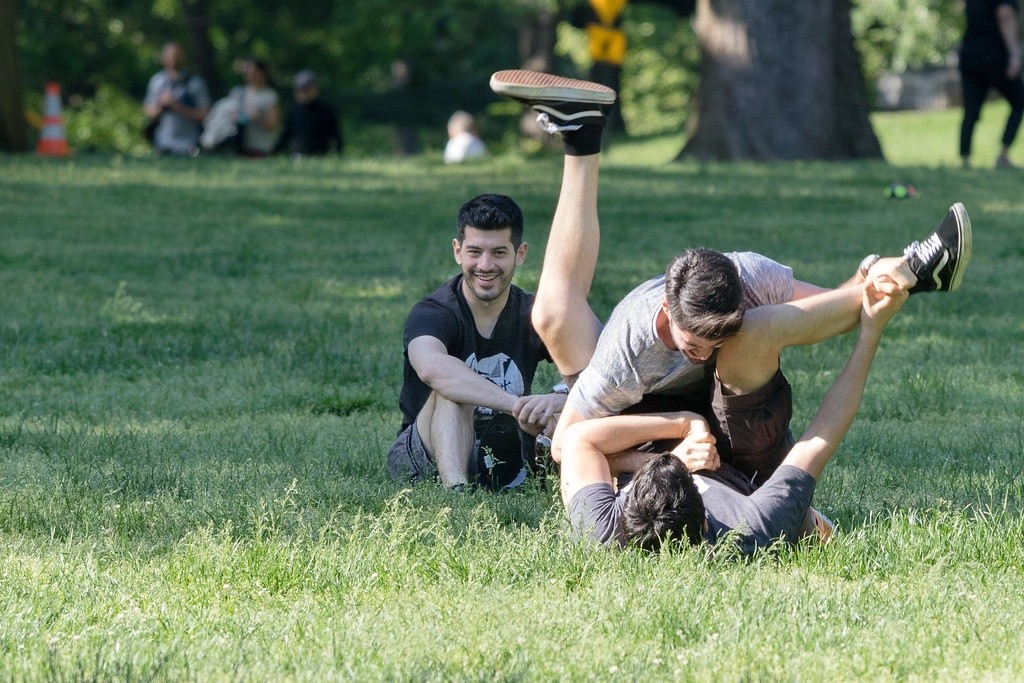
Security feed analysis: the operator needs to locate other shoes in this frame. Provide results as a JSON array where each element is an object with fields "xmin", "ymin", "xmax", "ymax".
[{"xmin": 808, "ymin": 504, "xmax": 838, "ymax": 548}]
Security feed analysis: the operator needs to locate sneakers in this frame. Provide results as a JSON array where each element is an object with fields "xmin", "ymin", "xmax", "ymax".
[
  {"xmin": 490, "ymin": 69, "xmax": 618, "ymax": 136},
  {"xmin": 903, "ymin": 201, "xmax": 973, "ymax": 293}
]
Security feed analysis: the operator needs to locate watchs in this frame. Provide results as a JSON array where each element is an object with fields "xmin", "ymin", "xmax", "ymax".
[
  {"xmin": 550, "ymin": 384, "xmax": 570, "ymax": 395},
  {"xmin": 858, "ymin": 253, "xmax": 881, "ymax": 279}
]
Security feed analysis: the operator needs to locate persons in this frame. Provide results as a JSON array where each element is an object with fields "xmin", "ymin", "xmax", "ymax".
[
  {"xmin": 444, "ymin": 111, "xmax": 489, "ymax": 162},
  {"xmin": 143, "ymin": 41, "xmax": 209, "ymax": 159},
  {"xmin": 272, "ymin": 69, "xmax": 343, "ymax": 162},
  {"xmin": 388, "ymin": 60, "xmax": 424, "ymax": 155},
  {"xmin": 386, "ymin": 194, "xmax": 581, "ymax": 502},
  {"xmin": 490, "ymin": 69, "xmax": 971, "ymax": 556},
  {"xmin": 957, "ymin": 1, "xmax": 1024, "ymax": 170},
  {"xmin": 549, "ymin": 247, "xmax": 920, "ymax": 545},
  {"xmin": 224, "ymin": 54, "xmax": 283, "ymax": 157}
]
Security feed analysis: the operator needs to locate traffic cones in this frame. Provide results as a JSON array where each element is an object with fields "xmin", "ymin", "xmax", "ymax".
[{"xmin": 31, "ymin": 83, "xmax": 72, "ymax": 158}]
